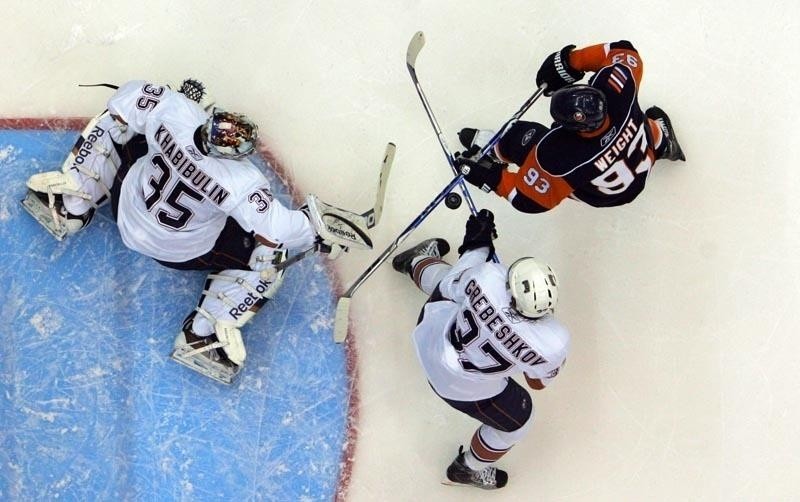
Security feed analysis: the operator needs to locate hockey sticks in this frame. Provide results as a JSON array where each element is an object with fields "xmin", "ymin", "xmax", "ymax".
[
  {"xmin": 406, "ymin": 31, "xmax": 500, "ymax": 264},
  {"xmin": 262, "ymin": 142, "xmax": 397, "ymax": 279},
  {"xmin": 333, "ymin": 84, "xmax": 549, "ymax": 346}
]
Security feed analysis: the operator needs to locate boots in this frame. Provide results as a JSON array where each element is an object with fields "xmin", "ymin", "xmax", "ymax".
[
  {"xmin": 392, "ymin": 238, "xmax": 450, "ymax": 274},
  {"xmin": 645, "ymin": 107, "xmax": 681, "ymax": 161},
  {"xmin": 441, "ymin": 445, "xmax": 508, "ymax": 491},
  {"xmin": 459, "ymin": 129, "xmax": 495, "ymax": 148},
  {"xmin": 27, "ymin": 189, "xmax": 95, "ymax": 234}
]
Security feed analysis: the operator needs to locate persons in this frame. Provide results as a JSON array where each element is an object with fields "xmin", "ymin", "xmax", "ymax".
[
  {"xmin": 393, "ymin": 209, "xmax": 571, "ymax": 489},
  {"xmin": 454, "ymin": 39, "xmax": 687, "ymax": 215},
  {"xmin": 19, "ymin": 82, "xmax": 348, "ymax": 386}
]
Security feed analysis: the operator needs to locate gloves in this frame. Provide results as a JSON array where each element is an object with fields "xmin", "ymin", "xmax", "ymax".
[
  {"xmin": 452, "ymin": 151, "xmax": 502, "ymax": 193},
  {"xmin": 537, "ymin": 45, "xmax": 585, "ymax": 97},
  {"xmin": 458, "ymin": 209, "xmax": 497, "ymax": 262}
]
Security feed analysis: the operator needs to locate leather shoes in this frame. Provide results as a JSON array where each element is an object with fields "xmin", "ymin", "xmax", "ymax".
[{"xmin": 174, "ymin": 329, "xmax": 242, "ymax": 376}]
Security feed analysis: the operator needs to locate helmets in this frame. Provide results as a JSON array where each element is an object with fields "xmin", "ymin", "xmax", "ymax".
[
  {"xmin": 550, "ymin": 84, "xmax": 608, "ymax": 132},
  {"xmin": 201, "ymin": 111, "xmax": 259, "ymax": 158},
  {"xmin": 506, "ymin": 257, "xmax": 558, "ymax": 319}
]
